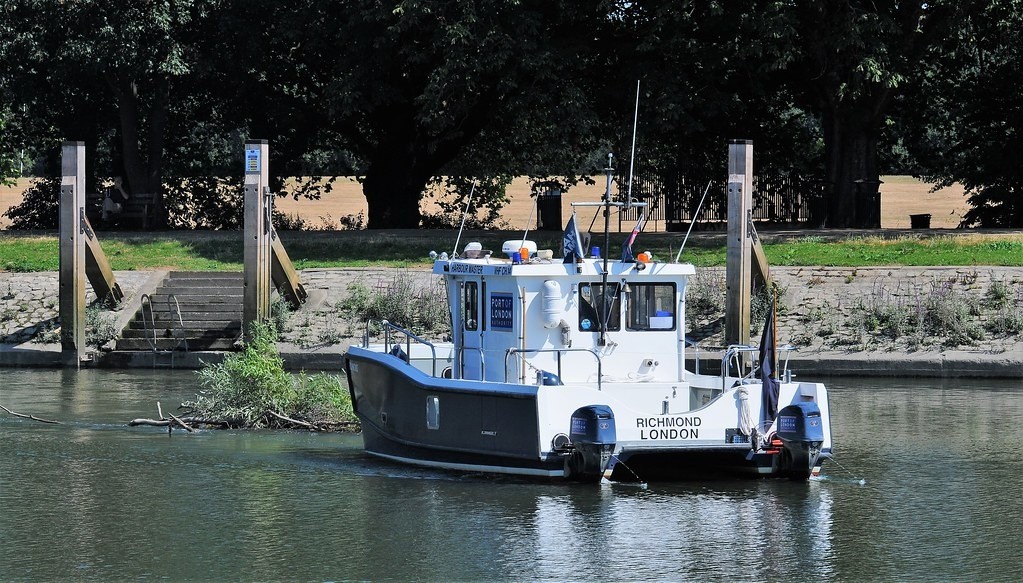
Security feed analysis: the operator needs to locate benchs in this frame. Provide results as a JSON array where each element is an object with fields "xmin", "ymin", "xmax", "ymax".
[{"xmin": 86, "ymin": 191, "xmax": 159, "ymax": 230}]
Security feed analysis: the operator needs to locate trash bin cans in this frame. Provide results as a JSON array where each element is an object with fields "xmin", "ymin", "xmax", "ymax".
[
  {"xmin": 537, "ymin": 182, "xmax": 562, "ymax": 230},
  {"xmin": 909, "ymin": 214, "xmax": 932, "ymax": 228},
  {"xmin": 853, "ymin": 179, "xmax": 884, "ymax": 228}
]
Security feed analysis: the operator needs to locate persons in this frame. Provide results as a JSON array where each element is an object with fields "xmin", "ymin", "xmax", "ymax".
[{"xmin": 595, "ymin": 290, "xmax": 618, "ymax": 327}]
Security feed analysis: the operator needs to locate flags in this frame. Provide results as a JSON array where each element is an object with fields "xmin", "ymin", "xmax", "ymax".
[
  {"xmin": 561, "ymin": 214, "xmax": 583, "ymax": 263},
  {"xmin": 620, "ymin": 218, "xmax": 640, "ymax": 263}
]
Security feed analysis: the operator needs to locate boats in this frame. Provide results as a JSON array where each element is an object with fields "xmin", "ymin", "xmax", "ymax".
[{"xmin": 334, "ymin": 73, "xmax": 835, "ymax": 488}]
